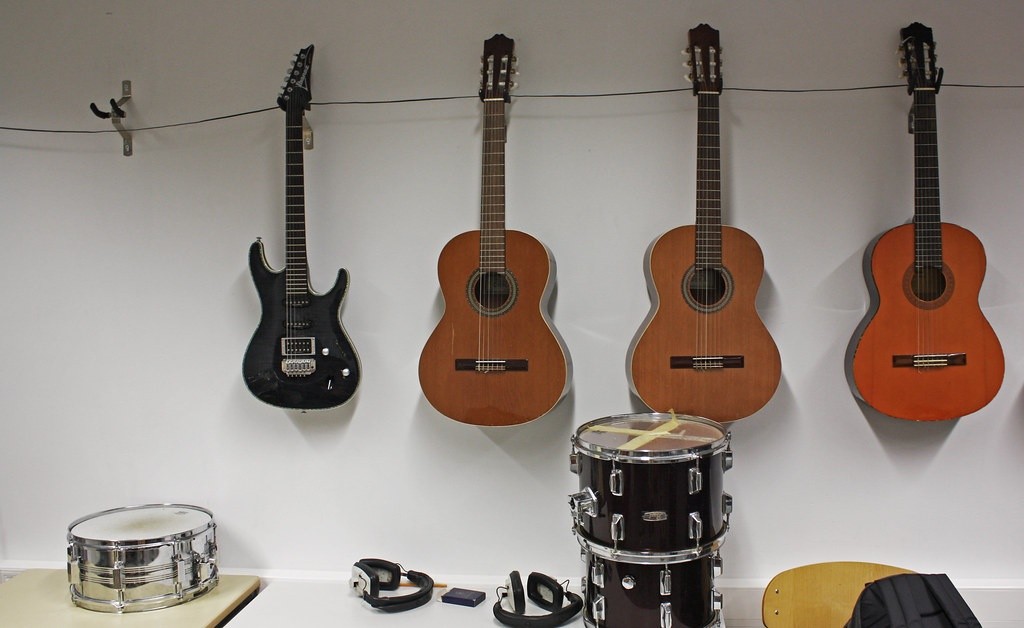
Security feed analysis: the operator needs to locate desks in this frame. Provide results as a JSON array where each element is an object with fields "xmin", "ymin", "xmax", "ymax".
[
  {"xmin": 224, "ymin": 578, "xmax": 1024, "ymax": 628},
  {"xmin": 0, "ymin": 568, "xmax": 260, "ymax": 628}
]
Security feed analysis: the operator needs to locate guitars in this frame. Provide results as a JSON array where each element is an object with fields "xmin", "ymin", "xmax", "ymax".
[
  {"xmin": 841, "ymin": 21, "xmax": 1008, "ymax": 421},
  {"xmin": 624, "ymin": 18, "xmax": 782, "ymax": 423},
  {"xmin": 417, "ymin": 32, "xmax": 577, "ymax": 428},
  {"xmin": 241, "ymin": 42, "xmax": 361, "ymax": 412}
]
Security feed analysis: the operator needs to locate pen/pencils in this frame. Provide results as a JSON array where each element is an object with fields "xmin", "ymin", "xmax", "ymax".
[{"xmin": 399, "ymin": 583, "xmax": 447, "ymax": 588}]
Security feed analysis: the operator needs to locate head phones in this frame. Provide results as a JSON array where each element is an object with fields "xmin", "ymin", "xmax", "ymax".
[
  {"xmin": 349, "ymin": 558, "xmax": 434, "ymax": 613},
  {"xmin": 493, "ymin": 571, "xmax": 583, "ymax": 628}
]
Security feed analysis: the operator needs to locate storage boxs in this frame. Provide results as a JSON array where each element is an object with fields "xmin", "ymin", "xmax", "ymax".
[{"xmin": 435, "ymin": 587, "xmax": 486, "ymax": 608}]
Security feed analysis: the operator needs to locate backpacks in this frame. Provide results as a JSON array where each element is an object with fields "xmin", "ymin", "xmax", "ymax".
[{"xmin": 844, "ymin": 573, "xmax": 982, "ymax": 628}]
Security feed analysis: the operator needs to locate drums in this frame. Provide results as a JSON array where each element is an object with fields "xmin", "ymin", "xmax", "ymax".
[
  {"xmin": 567, "ymin": 412, "xmax": 736, "ymax": 556},
  {"xmin": 582, "ymin": 552, "xmax": 725, "ymax": 628},
  {"xmin": 63, "ymin": 502, "xmax": 222, "ymax": 615}
]
